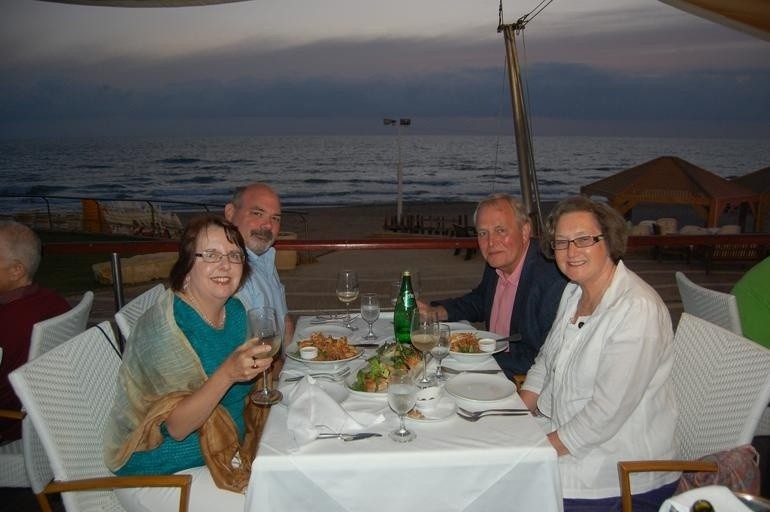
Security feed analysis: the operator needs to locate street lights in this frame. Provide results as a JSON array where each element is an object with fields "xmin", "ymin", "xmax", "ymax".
[{"xmin": 383, "ymin": 114, "xmax": 415, "ymax": 227}]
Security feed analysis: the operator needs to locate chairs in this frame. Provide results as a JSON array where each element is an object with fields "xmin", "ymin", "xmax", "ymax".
[
  {"xmin": 675, "ymin": 267, "xmax": 770, "ymax": 442},
  {"xmin": 1, "ymin": 288, "xmax": 97, "ymax": 511},
  {"xmin": 615, "ymin": 310, "xmax": 770, "ymax": 512},
  {"xmin": 112, "ymin": 278, "xmax": 169, "ymax": 344},
  {"xmin": 5, "ymin": 318, "xmax": 197, "ymax": 512}
]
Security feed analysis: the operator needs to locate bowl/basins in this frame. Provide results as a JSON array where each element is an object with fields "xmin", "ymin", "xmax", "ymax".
[
  {"xmin": 415, "ymin": 384, "xmax": 443, "ymax": 409},
  {"xmin": 299, "ymin": 345, "xmax": 319, "ymax": 359},
  {"xmin": 478, "ymin": 338, "xmax": 496, "ymax": 351}
]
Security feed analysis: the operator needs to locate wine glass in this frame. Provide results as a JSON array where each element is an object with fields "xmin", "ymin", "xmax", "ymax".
[
  {"xmin": 427, "ymin": 319, "xmax": 451, "ymax": 380},
  {"xmin": 357, "ymin": 293, "xmax": 382, "ymax": 340},
  {"xmin": 243, "ymin": 307, "xmax": 285, "ymax": 409},
  {"xmin": 335, "ymin": 270, "xmax": 360, "ymax": 330},
  {"xmin": 385, "ymin": 368, "xmax": 418, "ymax": 444},
  {"xmin": 408, "ymin": 304, "xmax": 440, "ymax": 387}
]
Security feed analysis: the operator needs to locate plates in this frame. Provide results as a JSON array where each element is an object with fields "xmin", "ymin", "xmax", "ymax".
[
  {"xmin": 344, "ymin": 364, "xmax": 389, "ymax": 397},
  {"xmin": 282, "ymin": 339, "xmax": 366, "ymax": 370},
  {"xmin": 443, "ymin": 370, "xmax": 518, "ymax": 403},
  {"xmin": 296, "ymin": 324, "xmax": 354, "ymax": 340},
  {"xmin": 444, "ymin": 328, "xmax": 509, "ymax": 363},
  {"xmin": 278, "ymin": 381, "xmax": 349, "ymax": 408},
  {"xmin": 401, "ymin": 395, "xmax": 455, "ymax": 423}
]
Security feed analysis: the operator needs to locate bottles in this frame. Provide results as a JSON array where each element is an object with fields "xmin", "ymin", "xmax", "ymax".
[{"xmin": 393, "ymin": 269, "xmax": 420, "ymax": 345}]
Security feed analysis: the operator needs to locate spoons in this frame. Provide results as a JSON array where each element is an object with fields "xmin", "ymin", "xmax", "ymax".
[{"xmin": 441, "ymin": 365, "xmax": 501, "ymax": 374}]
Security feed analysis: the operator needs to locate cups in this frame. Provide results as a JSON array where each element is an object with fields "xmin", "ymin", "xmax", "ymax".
[{"xmin": 390, "ymin": 282, "xmax": 402, "ymax": 306}]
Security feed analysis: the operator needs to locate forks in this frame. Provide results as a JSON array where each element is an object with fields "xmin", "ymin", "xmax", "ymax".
[
  {"xmin": 286, "ymin": 365, "xmax": 350, "ymax": 385},
  {"xmin": 457, "ymin": 406, "xmax": 533, "ymax": 421}
]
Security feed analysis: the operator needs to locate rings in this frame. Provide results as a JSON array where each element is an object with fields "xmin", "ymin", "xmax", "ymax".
[{"xmin": 251, "ymin": 359, "xmax": 258, "ymax": 370}]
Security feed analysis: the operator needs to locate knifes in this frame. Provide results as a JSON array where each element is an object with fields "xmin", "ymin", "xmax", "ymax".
[{"xmin": 316, "ymin": 431, "xmax": 383, "ymax": 441}]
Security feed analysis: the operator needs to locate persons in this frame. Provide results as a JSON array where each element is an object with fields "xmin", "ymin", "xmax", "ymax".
[
  {"xmin": 102, "ymin": 211, "xmax": 279, "ymax": 511},
  {"xmin": 516, "ymin": 197, "xmax": 683, "ymax": 511},
  {"xmin": 0, "ymin": 217, "xmax": 73, "ymax": 448},
  {"xmin": 222, "ymin": 181, "xmax": 296, "ymax": 382},
  {"xmin": 728, "ymin": 252, "xmax": 770, "ymax": 349},
  {"xmin": 413, "ymin": 191, "xmax": 569, "ymax": 385}
]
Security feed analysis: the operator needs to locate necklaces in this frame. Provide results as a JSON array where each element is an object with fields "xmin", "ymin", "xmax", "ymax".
[{"xmin": 183, "ymin": 289, "xmax": 228, "ymax": 331}]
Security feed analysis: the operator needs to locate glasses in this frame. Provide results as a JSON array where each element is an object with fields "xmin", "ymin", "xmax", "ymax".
[
  {"xmin": 550, "ymin": 234, "xmax": 606, "ymax": 251},
  {"xmin": 195, "ymin": 251, "xmax": 247, "ymax": 265}
]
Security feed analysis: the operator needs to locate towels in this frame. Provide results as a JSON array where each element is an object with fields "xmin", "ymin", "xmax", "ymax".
[{"xmin": 284, "ymin": 372, "xmax": 388, "ymax": 451}]
